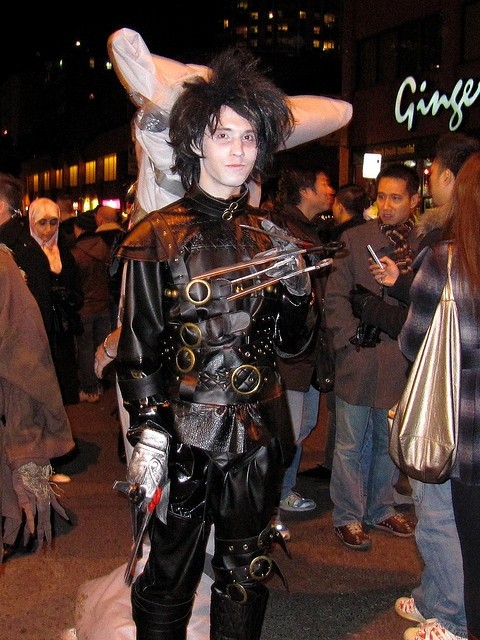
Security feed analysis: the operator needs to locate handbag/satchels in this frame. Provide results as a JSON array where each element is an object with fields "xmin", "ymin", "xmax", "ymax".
[
  {"xmin": 388, "ymin": 299, "xmax": 462, "ymax": 483},
  {"xmin": 52, "ymin": 301, "xmax": 85, "ymax": 339}
]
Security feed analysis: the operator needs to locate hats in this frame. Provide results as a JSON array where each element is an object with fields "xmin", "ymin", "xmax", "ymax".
[{"xmin": 73, "ymin": 212, "xmax": 96, "ymax": 231}]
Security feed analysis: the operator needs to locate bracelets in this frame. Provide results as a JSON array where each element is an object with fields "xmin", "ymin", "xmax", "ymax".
[{"xmin": 103, "ymin": 332, "xmax": 118, "ymax": 359}]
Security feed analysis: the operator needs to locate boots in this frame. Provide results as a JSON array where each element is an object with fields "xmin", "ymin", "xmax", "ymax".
[
  {"xmin": 129, "ymin": 574, "xmax": 195, "ymax": 640},
  {"xmin": 210, "ymin": 582, "xmax": 270, "ymax": 640}
]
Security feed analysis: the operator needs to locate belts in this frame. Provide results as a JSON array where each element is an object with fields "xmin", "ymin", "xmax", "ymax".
[{"xmin": 195, "ymin": 365, "xmax": 278, "ymax": 396}]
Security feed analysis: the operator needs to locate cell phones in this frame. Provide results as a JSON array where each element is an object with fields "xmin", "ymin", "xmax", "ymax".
[{"xmin": 364, "ymin": 243, "xmax": 390, "ymax": 274}]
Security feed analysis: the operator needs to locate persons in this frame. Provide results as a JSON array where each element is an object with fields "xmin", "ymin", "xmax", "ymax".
[
  {"xmin": 365, "ymin": 131, "xmax": 479, "ymax": 639},
  {"xmin": 395, "ymin": 151, "xmax": 479, "ymax": 638},
  {"xmin": 114, "ymin": 38, "xmax": 344, "ymax": 640},
  {"xmin": 55, "ymin": 190, "xmax": 79, "ymax": 249},
  {"xmin": 0, "ymin": 172, "xmax": 55, "ymax": 346},
  {"xmin": 262, "ymin": 158, "xmax": 336, "ymax": 540},
  {"xmin": 18, "ymin": 193, "xmax": 84, "ymax": 413},
  {"xmin": 91, "ymin": 206, "xmax": 129, "ymax": 249},
  {"xmin": 0, "ymin": 242, "xmax": 77, "ymax": 562},
  {"xmin": 298, "ymin": 391, "xmax": 332, "ymax": 487},
  {"xmin": 318, "ymin": 183, "xmax": 370, "ymax": 249},
  {"xmin": 324, "ymin": 165, "xmax": 417, "ymax": 549},
  {"xmin": 90, "ymin": 325, "xmax": 126, "ymax": 382},
  {"xmin": 65, "ymin": 214, "xmax": 112, "ymax": 407}
]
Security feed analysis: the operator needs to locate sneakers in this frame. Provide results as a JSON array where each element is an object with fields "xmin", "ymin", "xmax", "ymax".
[
  {"xmin": 394, "ymin": 597, "xmax": 423, "ymax": 623},
  {"xmin": 302, "ymin": 464, "xmax": 331, "ymax": 482},
  {"xmin": 376, "ymin": 511, "xmax": 416, "ymax": 538},
  {"xmin": 78, "ymin": 390, "xmax": 101, "ymax": 404},
  {"xmin": 403, "ymin": 617, "xmax": 468, "ymax": 640},
  {"xmin": 282, "ymin": 493, "xmax": 317, "ymax": 512},
  {"xmin": 333, "ymin": 522, "xmax": 373, "ymax": 550}
]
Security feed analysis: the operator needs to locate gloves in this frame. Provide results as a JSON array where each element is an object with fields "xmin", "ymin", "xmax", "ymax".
[
  {"xmin": 349, "ymin": 284, "xmax": 374, "ymax": 318},
  {"xmin": 253, "ymin": 219, "xmax": 313, "ymax": 297},
  {"xmin": 349, "ymin": 321, "xmax": 381, "ymax": 348},
  {"xmin": 126, "ymin": 429, "xmax": 173, "ymax": 526}
]
